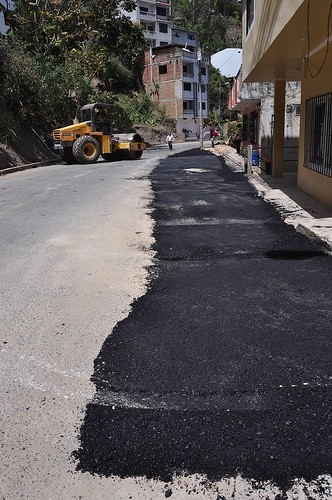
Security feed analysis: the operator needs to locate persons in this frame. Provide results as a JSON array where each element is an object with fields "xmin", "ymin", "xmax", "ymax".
[
  {"xmin": 166, "ymin": 132, "xmax": 174, "ymax": 150},
  {"xmin": 96, "ymin": 108, "xmax": 104, "ymax": 127},
  {"xmin": 235, "ymin": 124, "xmax": 242, "ymax": 153}
]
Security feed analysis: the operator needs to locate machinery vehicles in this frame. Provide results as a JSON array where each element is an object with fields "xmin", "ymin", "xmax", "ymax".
[{"xmin": 52, "ymin": 102, "xmax": 144, "ymax": 162}]
